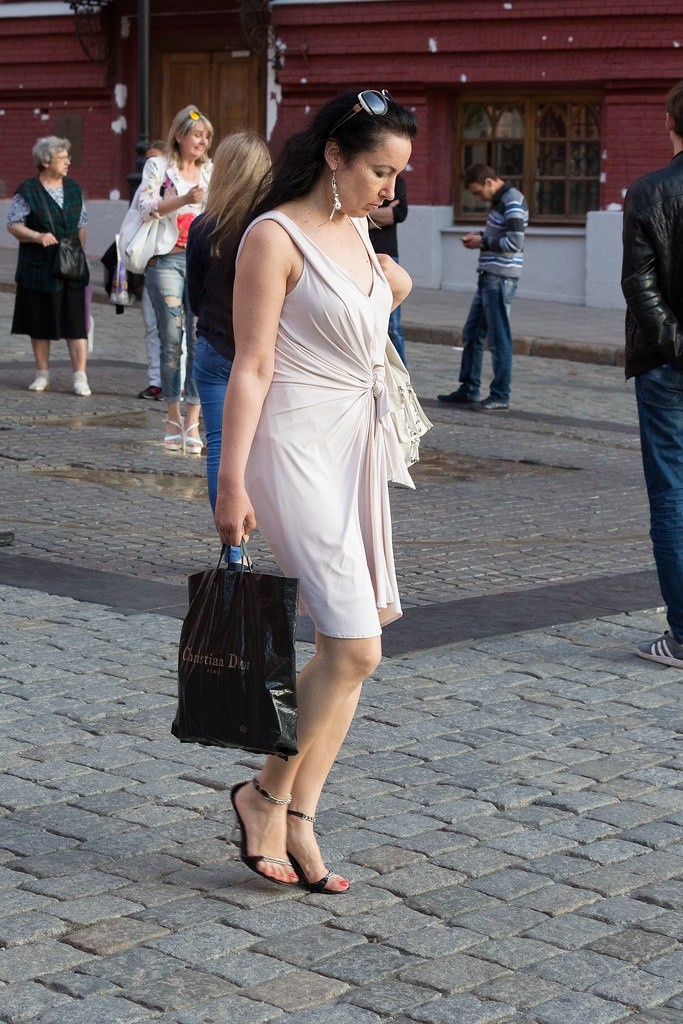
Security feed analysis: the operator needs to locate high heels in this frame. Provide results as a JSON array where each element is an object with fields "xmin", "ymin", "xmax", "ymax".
[
  {"xmin": 163, "ymin": 418, "xmax": 183, "ymax": 451},
  {"xmin": 230, "ymin": 778, "xmax": 297, "ymax": 887},
  {"xmin": 184, "ymin": 420, "xmax": 204, "ymax": 454},
  {"xmin": 285, "ymin": 809, "xmax": 350, "ymax": 895}
]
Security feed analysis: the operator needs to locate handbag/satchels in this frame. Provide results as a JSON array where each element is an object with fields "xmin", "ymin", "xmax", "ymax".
[
  {"xmin": 59, "ymin": 238, "xmax": 87, "ymax": 279},
  {"xmin": 111, "ymin": 235, "xmax": 129, "ymax": 306},
  {"xmin": 172, "ymin": 541, "xmax": 299, "ymax": 761},
  {"xmin": 116, "ymin": 159, "xmax": 162, "ymax": 274}
]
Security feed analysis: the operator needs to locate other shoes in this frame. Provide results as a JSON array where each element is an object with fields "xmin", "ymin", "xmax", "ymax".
[
  {"xmin": 438, "ymin": 390, "xmax": 476, "ymax": 404},
  {"xmin": 73, "ymin": 372, "xmax": 92, "ymax": 396},
  {"xmin": 477, "ymin": 395, "xmax": 510, "ymax": 409}
]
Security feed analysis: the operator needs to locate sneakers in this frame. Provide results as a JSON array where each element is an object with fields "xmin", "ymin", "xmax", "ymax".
[
  {"xmin": 138, "ymin": 386, "xmax": 161, "ymax": 399},
  {"xmin": 28, "ymin": 373, "xmax": 50, "ymax": 390},
  {"xmin": 636, "ymin": 631, "xmax": 683, "ymax": 669},
  {"xmin": 155, "ymin": 393, "xmax": 165, "ymax": 401}
]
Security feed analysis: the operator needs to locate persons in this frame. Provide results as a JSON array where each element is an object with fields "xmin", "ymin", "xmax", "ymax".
[
  {"xmin": 187, "ymin": 133, "xmax": 273, "ymax": 572},
  {"xmin": 620, "ymin": 85, "xmax": 683, "ymax": 669},
  {"xmin": 7, "ymin": 137, "xmax": 91, "ymax": 395},
  {"xmin": 367, "ymin": 176, "xmax": 408, "ymax": 368},
  {"xmin": 214, "ymin": 98, "xmax": 412, "ymax": 893},
  {"xmin": 139, "ymin": 141, "xmax": 188, "ymax": 401},
  {"xmin": 139, "ymin": 106, "xmax": 214, "ymax": 453},
  {"xmin": 438, "ymin": 162, "xmax": 529, "ymax": 412}
]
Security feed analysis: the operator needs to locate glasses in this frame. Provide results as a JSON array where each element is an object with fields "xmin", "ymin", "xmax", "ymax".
[
  {"xmin": 53, "ymin": 156, "xmax": 71, "ymax": 161},
  {"xmin": 329, "ymin": 89, "xmax": 396, "ymax": 134},
  {"xmin": 178, "ymin": 110, "xmax": 209, "ymax": 129}
]
